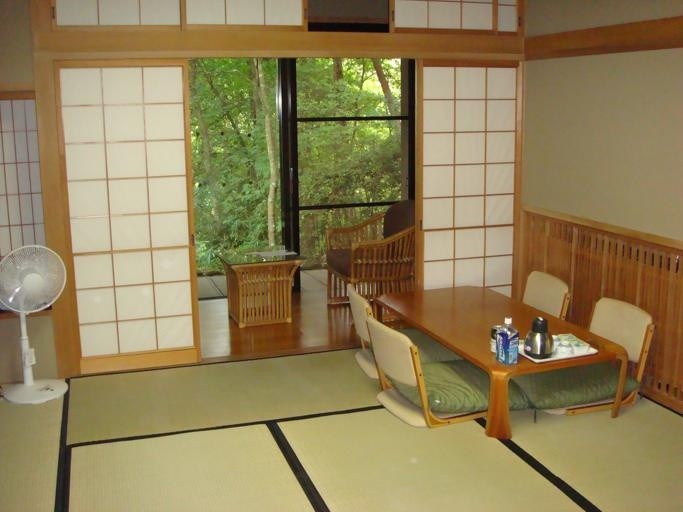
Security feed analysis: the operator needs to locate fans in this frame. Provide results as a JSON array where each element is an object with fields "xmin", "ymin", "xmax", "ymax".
[{"xmin": 0, "ymin": 244, "xmax": 69, "ymax": 405}]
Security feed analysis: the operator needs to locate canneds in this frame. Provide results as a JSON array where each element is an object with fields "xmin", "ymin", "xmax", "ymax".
[{"xmin": 491, "ymin": 325, "xmax": 500, "ymax": 353}]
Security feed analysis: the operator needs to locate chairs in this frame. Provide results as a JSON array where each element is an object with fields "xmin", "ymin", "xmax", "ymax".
[
  {"xmin": 346, "ymin": 283, "xmax": 463, "ymax": 380},
  {"xmin": 326, "ymin": 201, "xmax": 417, "ymax": 322},
  {"xmin": 522, "ymin": 270, "xmax": 572, "ymax": 322},
  {"xmin": 366, "ymin": 317, "xmax": 531, "ymax": 429},
  {"xmin": 511, "ymin": 297, "xmax": 655, "ymax": 418}
]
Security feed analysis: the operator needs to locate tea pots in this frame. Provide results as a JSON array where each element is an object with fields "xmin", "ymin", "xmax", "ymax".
[{"xmin": 524, "ymin": 315, "xmax": 556, "ymax": 359}]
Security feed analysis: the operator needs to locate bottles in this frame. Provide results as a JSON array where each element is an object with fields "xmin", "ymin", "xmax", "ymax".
[{"xmin": 496, "ymin": 316, "xmax": 518, "ymax": 364}]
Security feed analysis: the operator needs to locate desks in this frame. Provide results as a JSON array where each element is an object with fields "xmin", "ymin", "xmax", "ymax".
[{"xmin": 373, "ymin": 286, "xmax": 629, "ymax": 440}]
